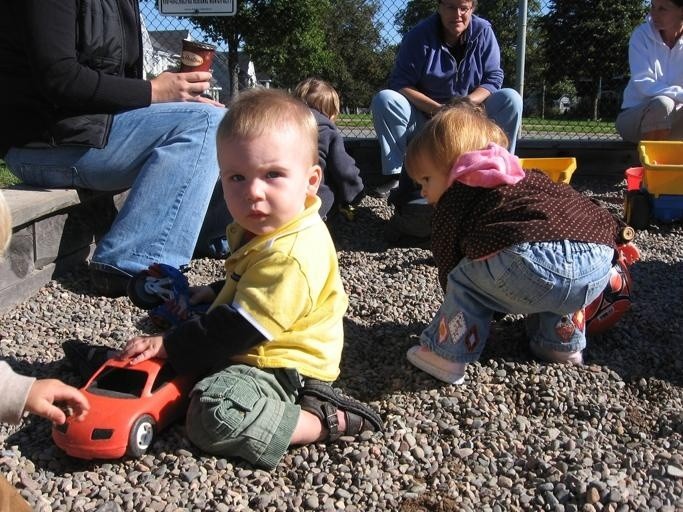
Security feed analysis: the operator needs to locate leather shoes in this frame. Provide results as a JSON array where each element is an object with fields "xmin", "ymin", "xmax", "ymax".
[{"xmin": 373, "ymin": 178, "xmax": 401, "ymax": 198}]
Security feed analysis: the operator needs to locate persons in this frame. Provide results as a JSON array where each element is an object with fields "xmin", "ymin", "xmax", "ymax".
[
  {"xmin": 291, "ymin": 76, "xmax": 365, "ymax": 230},
  {"xmin": 368, "ymin": 1, "xmax": 523, "ymax": 198},
  {"xmin": 616, "ymin": 0, "xmax": 682, "ymax": 165},
  {"xmin": 401, "ymin": 95, "xmax": 643, "ymax": 384},
  {"xmin": 0, "ymin": 0, "xmax": 232, "ymax": 296},
  {"xmin": 0, "ymin": 187, "xmax": 92, "ymax": 427},
  {"xmin": 116, "ymin": 83, "xmax": 386, "ymax": 473}
]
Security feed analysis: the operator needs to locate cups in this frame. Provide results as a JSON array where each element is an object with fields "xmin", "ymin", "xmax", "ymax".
[{"xmin": 180, "ymin": 38, "xmax": 216, "ymax": 95}]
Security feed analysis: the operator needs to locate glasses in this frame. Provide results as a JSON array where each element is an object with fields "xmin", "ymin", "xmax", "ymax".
[{"xmin": 439, "ymin": 5, "xmax": 473, "ymax": 15}]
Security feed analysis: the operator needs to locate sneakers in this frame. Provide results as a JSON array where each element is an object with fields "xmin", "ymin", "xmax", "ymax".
[
  {"xmin": 529, "ymin": 338, "xmax": 584, "ymax": 366},
  {"xmin": 405, "ymin": 344, "xmax": 466, "ymax": 385}
]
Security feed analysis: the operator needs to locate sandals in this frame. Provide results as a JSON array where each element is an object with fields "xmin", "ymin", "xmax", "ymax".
[{"xmin": 297, "ymin": 377, "xmax": 385, "ymax": 445}]
